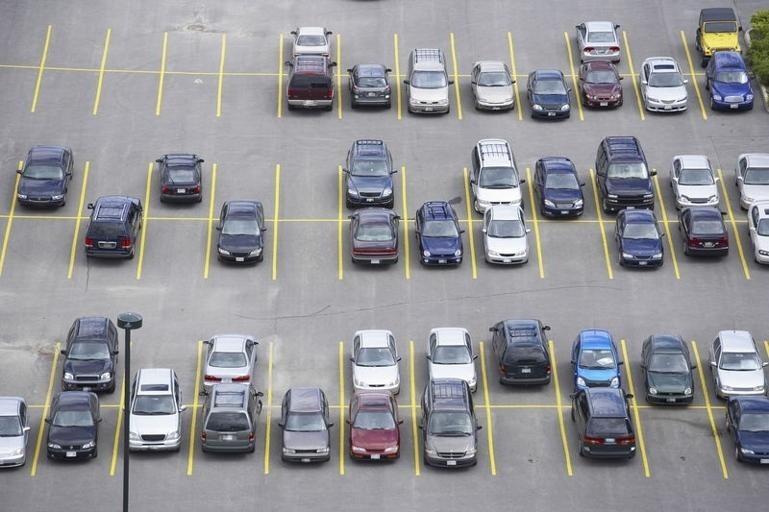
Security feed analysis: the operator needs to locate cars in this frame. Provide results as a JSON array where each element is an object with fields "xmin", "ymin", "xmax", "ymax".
[
  {"xmin": 569, "ymin": 324, "xmax": 769, "ymax": 472},
  {"xmin": 678, "ymin": 206, "xmax": 730, "ymax": 258},
  {"xmin": 348, "ymin": 328, "xmax": 399, "ymax": 394},
  {"xmin": 413, "ymin": 201, "xmax": 463, "ymax": 267},
  {"xmin": 16, "ymin": 145, "xmax": 72, "ymax": 208},
  {"xmin": 198, "ymin": 383, "xmax": 260, "ymax": 454},
  {"xmin": 84, "ymin": 194, "xmax": 140, "ymax": 260},
  {"xmin": 669, "ymin": 155, "xmax": 721, "ymax": 211},
  {"xmin": 469, "ymin": 59, "xmax": 517, "ymax": 112},
  {"xmin": 44, "ymin": 392, "xmax": 101, "ymax": 461},
  {"xmin": 614, "ymin": 209, "xmax": 665, "ymax": 269},
  {"xmin": 279, "ymin": 385, "xmax": 333, "ymax": 463},
  {"xmin": 155, "ymin": 151, "xmax": 205, "ymax": 205},
  {"xmin": 534, "ymin": 156, "xmax": 586, "ymax": 220},
  {"xmin": 214, "ymin": 199, "xmax": 266, "ymax": 264},
  {"xmin": 487, "ymin": 317, "xmax": 552, "ymax": 385},
  {"xmin": 747, "ymin": 199, "xmax": 769, "ymax": 266},
  {"xmin": 288, "ymin": 25, "xmax": 332, "ymax": 57},
  {"xmin": 349, "ymin": 395, "xmax": 400, "ymax": 462},
  {"xmin": 347, "ymin": 208, "xmax": 400, "ymax": 267},
  {"xmin": 1, "ymin": 396, "xmax": 30, "ymax": 468},
  {"xmin": 204, "ymin": 331, "xmax": 258, "ymax": 386},
  {"xmin": 733, "ymin": 152, "xmax": 769, "ymax": 211},
  {"xmin": 403, "ymin": 47, "xmax": 451, "ymax": 117},
  {"xmin": 340, "ymin": 139, "xmax": 397, "ymax": 208},
  {"xmin": 429, "ymin": 327, "xmax": 481, "ymax": 392},
  {"xmin": 62, "ymin": 315, "xmax": 119, "ymax": 391},
  {"xmin": 422, "ymin": 379, "xmax": 479, "ymax": 469},
  {"xmin": 128, "ymin": 368, "xmax": 185, "ymax": 449},
  {"xmin": 481, "ymin": 204, "xmax": 532, "ymax": 266},
  {"xmin": 346, "ymin": 61, "xmax": 392, "ymax": 109},
  {"xmin": 526, "ymin": 3, "xmax": 757, "ymax": 119}
]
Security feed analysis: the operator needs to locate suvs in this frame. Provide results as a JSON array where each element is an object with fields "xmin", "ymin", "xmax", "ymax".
[
  {"xmin": 468, "ymin": 138, "xmax": 526, "ymax": 215},
  {"xmin": 594, "ymin": 138, "xmax": 658, "ymax": 215}
]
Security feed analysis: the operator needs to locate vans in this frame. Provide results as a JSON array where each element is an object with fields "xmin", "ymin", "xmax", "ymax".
[{"xmin": 284, "ymin": 54, "xmax": 338, "ymax": 112}]
